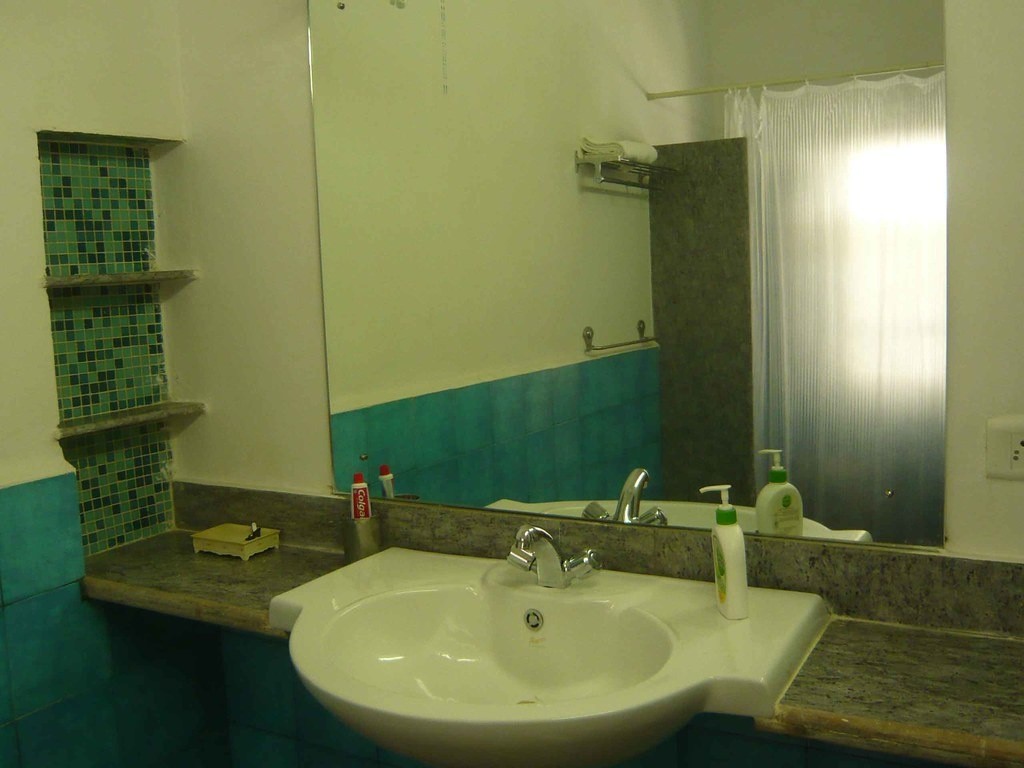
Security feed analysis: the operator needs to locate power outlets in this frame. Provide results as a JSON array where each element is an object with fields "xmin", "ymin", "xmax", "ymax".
[{"xmin": 983, "ymin": 413, "xmax": 1024, "ymax": 482}]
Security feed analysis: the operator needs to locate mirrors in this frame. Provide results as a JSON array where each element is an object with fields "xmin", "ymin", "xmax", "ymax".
[{"xmin": 305, "ymin": 0, "xmax": 950, "ymax": 549}]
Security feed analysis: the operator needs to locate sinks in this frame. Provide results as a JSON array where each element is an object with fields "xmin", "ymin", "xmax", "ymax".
[
  {"xmin": 267, "ymin": 543, "xmax": 832, "ymax": 768},
  {"xmin": 483, "ymin": 496, "xmax": 876, "ymax": 548}
]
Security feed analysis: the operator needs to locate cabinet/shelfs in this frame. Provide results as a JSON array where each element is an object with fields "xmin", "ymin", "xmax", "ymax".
[{"xmin": 37, "ymin": 126, "xmax": 209, "ymax": 444}]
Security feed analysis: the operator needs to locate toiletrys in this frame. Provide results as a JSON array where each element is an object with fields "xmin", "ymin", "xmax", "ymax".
[
  {"xmin": 377, "ymin": 466, "xmax": 397, "ymax": 501},
  {"xmin": 348, "ymin": 473, "xmax": 373, "ymax": 520},
  {"xmin": 754, "ymin": 448, "xmax": 809, "ymax": 538},
  {"xmin": 698, "ymin": 484, "xmax": 752, "ymax": 621}
]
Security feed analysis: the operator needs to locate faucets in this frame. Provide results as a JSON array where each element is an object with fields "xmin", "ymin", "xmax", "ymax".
[
  {"xmin": 506, "ymin": 526, "xmax": 573, "ymax": 590},
  {"xmin": 614, "ymin": 467, "xmax": 650, "ymax": 525}
]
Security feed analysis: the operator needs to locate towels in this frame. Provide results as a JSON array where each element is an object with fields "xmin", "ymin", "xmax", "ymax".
[{"xmin": 581, "ymin": 135, "xmax": 660, "ymax": 166}]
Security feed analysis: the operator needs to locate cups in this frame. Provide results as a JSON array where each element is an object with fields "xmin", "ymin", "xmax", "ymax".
[{"xmin": 340, "ymin": 509, "xmax": 383, "ymax": 562}]
